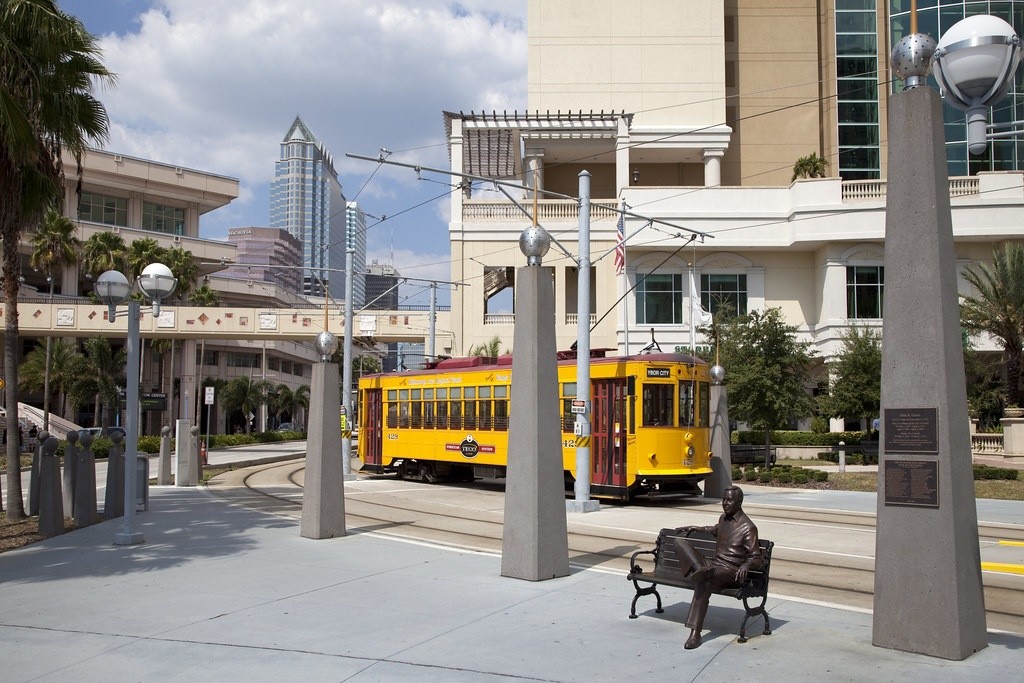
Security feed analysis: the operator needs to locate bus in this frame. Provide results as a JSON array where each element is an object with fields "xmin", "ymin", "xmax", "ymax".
[{"xmin": 355, "ymin": 329, "xmax": 712, "ymax": 503}]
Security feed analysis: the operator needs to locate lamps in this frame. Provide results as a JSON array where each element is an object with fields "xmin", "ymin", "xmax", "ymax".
[{"xmin": 634, "ymin": 167, "xmax": 640, "ymax": 184}]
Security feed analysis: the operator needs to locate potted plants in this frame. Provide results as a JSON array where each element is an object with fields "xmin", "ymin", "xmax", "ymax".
[{"xmin": 1004, "ymin": 402, "xmax": 1024, "ymax": 418}]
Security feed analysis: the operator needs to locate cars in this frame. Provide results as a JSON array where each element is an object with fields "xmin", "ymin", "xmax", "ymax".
[
  {"xmin": 277, "ymin": 422, "xmax": 293, "ymax": 431},
  {"xmin": 76, "ymin": 427, "xmax": 126, "ymax": 443}
]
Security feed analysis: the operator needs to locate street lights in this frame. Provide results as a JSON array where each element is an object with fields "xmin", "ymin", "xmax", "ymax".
[
  {"xmin": 94, "ymin": 263, "xmax": 178, "ymax": 545},
  {"xmin": 870, "ymin": 14, "xmax": 1024, "ymax": 661}
]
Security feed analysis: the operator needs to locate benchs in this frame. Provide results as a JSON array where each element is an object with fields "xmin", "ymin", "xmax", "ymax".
[
  {"xmin": 627, "ymin": 528, "xmax": 775, "ymax": 643},
  {"xmin": 861, "ymin": 440, "xmax": 878, "ymax": 467},
  {"xmin": 730, "ymin": 444, "xmax": 777, "ymax": 467}
]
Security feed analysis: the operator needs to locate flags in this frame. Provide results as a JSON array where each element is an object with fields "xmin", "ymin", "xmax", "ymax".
[
  {"xmin": 614, "ymin": 212, "xmax": 625, "ymax": 276},
  {"xmin": 691, "ymin": 273, "xmax": 712, "ymax": 327}
]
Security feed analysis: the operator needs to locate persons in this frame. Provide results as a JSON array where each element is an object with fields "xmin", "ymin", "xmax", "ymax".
[{"xmin": 673, "ymin": 486, "xmax": 767, "ymax": 649}]
